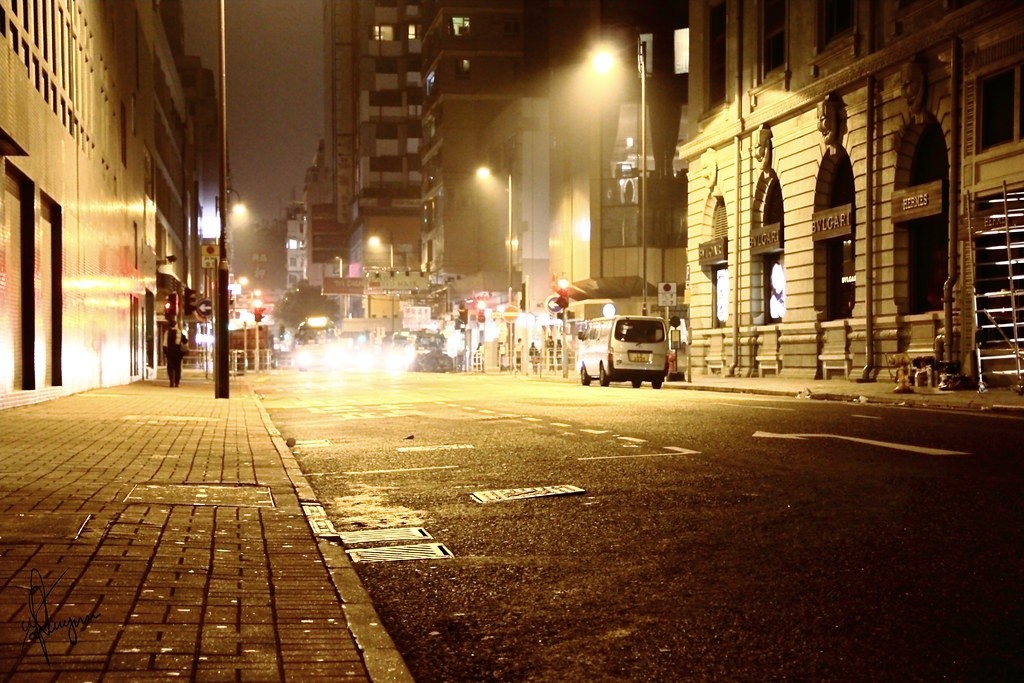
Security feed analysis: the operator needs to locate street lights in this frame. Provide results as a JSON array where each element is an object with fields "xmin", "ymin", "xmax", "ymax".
[
  {"xmin": 239, "ymin": 277, "xmax": 248, "ymax": 375},
  {"xmin": 369, "ymin": 236, "xmax": 395, "ymax": 336},
  {"xmin": 478, "ymin": 167, "xmax": 512, "ymax": 375},
  {"xmin": 589, "ymin": 33, "xmax": 647, "ymax": 312},
  {"xmin": 251, "ymin": 289, "xmax": 259, "ymax": 371}
]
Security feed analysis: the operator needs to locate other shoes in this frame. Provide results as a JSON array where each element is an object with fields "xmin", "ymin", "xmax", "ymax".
[
  {"xmin": 175, "ymin": 384, "xmax": 181, "ymax": 387},
  {"xmin": 170, "ymin": 383, "xmax": 174, "ymax": 387}
]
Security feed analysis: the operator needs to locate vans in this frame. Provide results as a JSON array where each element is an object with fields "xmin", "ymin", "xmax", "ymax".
[{"xmin": 574, "ymin": 315, "xmax": 668, "ymax": 386}]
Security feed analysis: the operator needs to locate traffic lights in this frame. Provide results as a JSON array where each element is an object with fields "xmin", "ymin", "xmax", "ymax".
[
  {"xmin": 184, "ymin": 288, "xmax": 197, "ymax": 316},
  {"xmin": 254, "ymin": 307, "xmax": 265, "ymax": 323}
]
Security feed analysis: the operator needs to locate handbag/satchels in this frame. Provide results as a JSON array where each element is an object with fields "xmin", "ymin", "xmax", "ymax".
[{"xmin": 179, "ymin": 342, "xmax": 189, "ymax": 356}]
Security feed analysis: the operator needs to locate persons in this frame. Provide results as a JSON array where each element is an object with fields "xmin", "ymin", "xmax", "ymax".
[
  {"xmin": 514, "ymin": 337, "xmax": 524, "ymax": 371},
  {"xmin": 546, "ymin": 336, "xmax": 554, "ymax": 370},
  {"xmin": 556, "ymin": 339, "xmax": 562, "ymax": 370},
  {"xmin": 476, "ymin": 342, "xmax": 481, "ymax": 350},
  {"xmin": 163, "ymin": 320, "xmax": 188, "ymax": 388},
  {"xmin": 529, "ymin": 342, "xmax": 540, "ymax": 374}
]
